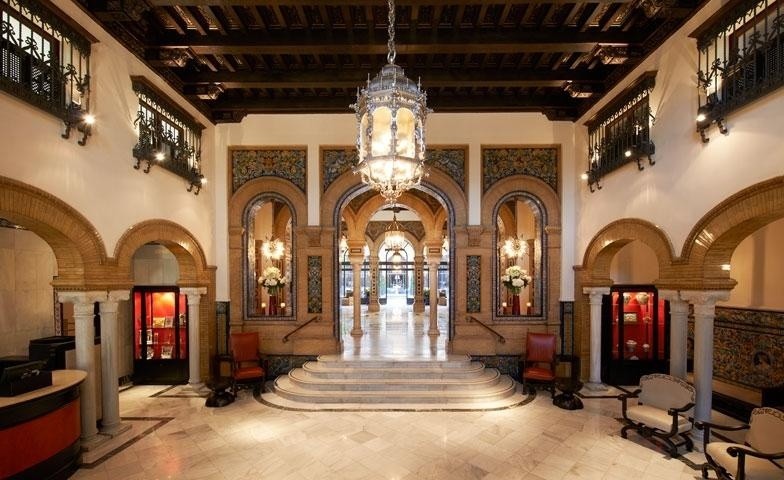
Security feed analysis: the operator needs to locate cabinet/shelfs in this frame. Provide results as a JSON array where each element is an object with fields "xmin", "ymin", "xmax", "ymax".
[
  {"xmin": 131, "ymin": 285, "xmax": 190, "ymax": 385},
  {"xmin": 601, "ymin": 283, "xmax": 670, "ymax": 385}
]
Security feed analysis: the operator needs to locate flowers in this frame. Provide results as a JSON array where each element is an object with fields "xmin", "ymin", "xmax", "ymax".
[
  {"xmin": 501, "ymin": 265, "xmax": 532, "ymax": 296},
  {"xmin": 258, "ymin": 266, "xmax": 285, "ymax": 296}
]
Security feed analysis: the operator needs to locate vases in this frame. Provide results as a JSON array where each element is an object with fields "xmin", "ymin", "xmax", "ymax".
[
  {"xmin": 512, "ymin": 296, "xmax": 520, "ymax": 315},
  {"xmin": 269, "ymin": 296, "xmax": 277, "ymax": 315}
]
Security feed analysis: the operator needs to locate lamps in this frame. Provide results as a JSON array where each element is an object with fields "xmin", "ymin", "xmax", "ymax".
[
  {"xmin": 384, "ymin": 210, "xmax": 405, "ymax": 249},
  {"xmin": 349, "ymin": 0, "xmax": 428, "ymax": 208}
]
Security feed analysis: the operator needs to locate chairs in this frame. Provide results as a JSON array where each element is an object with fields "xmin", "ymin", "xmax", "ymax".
[
  {"xmin": 521, "ymin": 332, "xmax": 556, "ymax": 394},
  {"xmin": 228, "ymin": 332, "xmax": 265, "ymax": 395},
  {"xmin": 617, "ymin": 374, "xmax": 696, "ymax": 458},
  {"xmin": 695, "ymin": 406, "xmax": 784, "ymax": 480}
]
